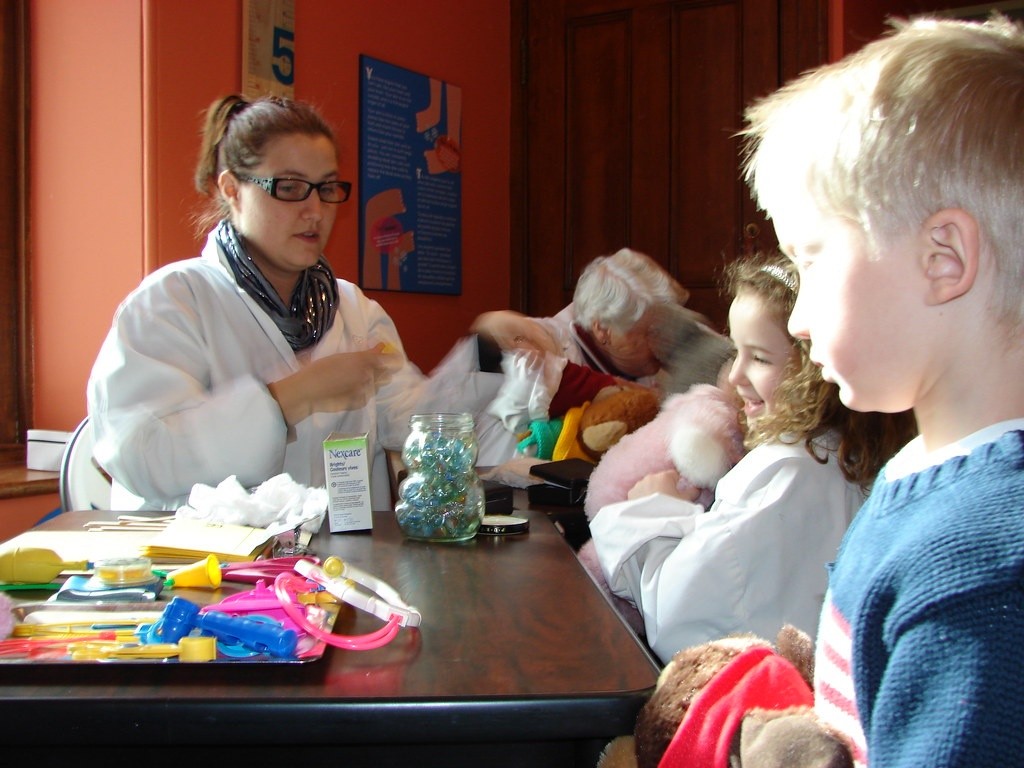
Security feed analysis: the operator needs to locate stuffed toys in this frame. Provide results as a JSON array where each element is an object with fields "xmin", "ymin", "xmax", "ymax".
[
  {"xmin": 516, "ymin": 314, "xmax": 751, "ymax": 520},
  {"xmin": 592, "ymin": 619, "xmax": 853, "ymax": 768}
]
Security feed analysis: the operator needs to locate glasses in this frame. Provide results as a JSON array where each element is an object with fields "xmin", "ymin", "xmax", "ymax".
[{"xmin": 231, "ymin": 171, "xmax": 352, "ymax": 203}]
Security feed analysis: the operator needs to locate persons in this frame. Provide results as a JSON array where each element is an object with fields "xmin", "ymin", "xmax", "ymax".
[
  {"xmin": 478, "ymin": 242, "xmax": 685, "ymax": 541},
  {"xmin": 83, "ymin": 89, "xmax": 564, "ymax": 510},
  {"xmin": 732, "ymin": 18, "xmax": 1020, "ymax": 767},
  {"xmin": 592, "ymin": 246, "xmax": 875, "ymax": 669}
]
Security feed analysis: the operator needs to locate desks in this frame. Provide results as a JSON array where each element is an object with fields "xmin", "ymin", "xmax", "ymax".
[{"xmin": 0, "ymin": 510, "xmax": 660, "ymax": 767}]
